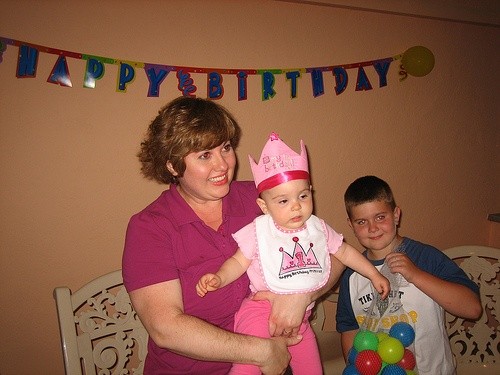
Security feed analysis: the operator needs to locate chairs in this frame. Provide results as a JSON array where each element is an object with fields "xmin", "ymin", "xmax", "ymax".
[
  {"xmin": 441, "ymin": 246, "xmax": 500, "ymax": 375},
  {"xmin": 53, "ymin": 269, "xmax": 149, "ymax": 375}
]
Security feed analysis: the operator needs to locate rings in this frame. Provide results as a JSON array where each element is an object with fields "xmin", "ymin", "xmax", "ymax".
[{"xmin": 284, "ymin": 330, "xmax": 292, "ymax": 334}]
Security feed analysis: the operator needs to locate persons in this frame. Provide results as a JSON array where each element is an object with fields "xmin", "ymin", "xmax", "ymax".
[
  {"xmin": 196, "ymin": 130, "xmax": 391, "ymax": 375},
  {"xmin": 122, "ymin": 95, "xmax": 347, "ymax": 375},
  {"xmin": 335, "ymin": 176, "xmax": 484, "ymax": 375}
]
